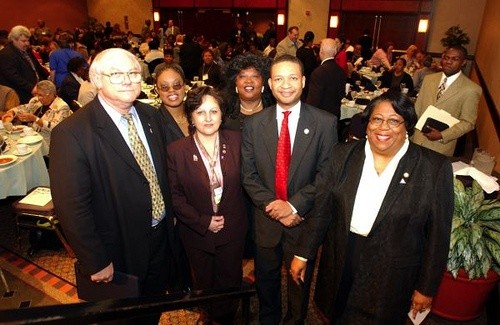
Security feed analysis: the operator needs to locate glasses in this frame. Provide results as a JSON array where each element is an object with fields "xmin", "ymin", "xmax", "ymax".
[
  {"xmin": 158, "ymin": 83, "xmax": 184, "ymax": 91},
  {"xmin": 101, "ymin": 72, "xmax": 141, "ymax": 84},
  {"xmin": 369, "ymin": 115, "xmax": 405, "ymax": 127}
]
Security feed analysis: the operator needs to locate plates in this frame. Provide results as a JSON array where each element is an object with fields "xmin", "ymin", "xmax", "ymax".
[
  {"xmin": 0, "ymin": 154, "xmax": 17, "ymax": 166},
  {"xmin": 11, "ymin": 125, "xmax": 27, "ymax": 133},
  {"xmin": 12, "ymin": 149, "xmax": 32, "ymax": 156},
  {"xmin": 17, "ymin": 135, "xmax": 43, "ymax": 145}
]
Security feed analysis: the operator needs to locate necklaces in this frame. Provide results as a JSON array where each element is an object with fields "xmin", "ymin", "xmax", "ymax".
[{"xmin": 238, "ymin": 101, "xmax": 263, "ymax": 112}]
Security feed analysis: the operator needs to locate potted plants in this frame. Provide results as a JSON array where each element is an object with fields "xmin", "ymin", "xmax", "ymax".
[{"xmin": 431, "ymin": 175, "xmax": 500, "ymax": 321}]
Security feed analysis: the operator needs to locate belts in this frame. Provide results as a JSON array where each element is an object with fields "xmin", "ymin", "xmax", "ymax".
[{"xmin": 151, "ymin": 220, "xmax": 164, "ymax": 231}]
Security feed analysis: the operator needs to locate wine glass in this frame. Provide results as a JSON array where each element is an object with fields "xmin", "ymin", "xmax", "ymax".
[
  {"xmin": 374, "ymin": 80, "xmax": 381, "ymax": 90},
  {"xmin": 400, "ymin": 83, "xmax": 406, "ymax": 93}
]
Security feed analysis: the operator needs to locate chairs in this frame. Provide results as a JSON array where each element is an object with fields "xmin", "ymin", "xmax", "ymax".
[
  {"xmin": 10, "ymin": 184, "xmax": 78, "ymax": 259},
  {"xmin": 0, "ymin": 119, "xmax": 48, "ymax": 225}
]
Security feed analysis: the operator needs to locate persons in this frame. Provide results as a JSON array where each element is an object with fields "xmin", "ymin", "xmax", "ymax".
[
  {"xmin": 166, "ymin": 87, "xmax": 251, "ymax": 295},
  {"xmin": 152, "ymin": 60, "xmax": 195, "ymax": 144},
  {"xmin": 409, "ymin": 45, "xmax": 482, "ymax": 157},
  {"xmin": 241, "ymin": 54, "xmax": 337, "ymax": 325},
  {"xmin": 220, "ymin": 54, "xmax": 278, "ymax": 130},
  {"xmin": 0, "ymin": 20, "xmax": 441, "ymax": 153},
  {"xmin": 292, "ymin": 93, "xmax": 454, "ymax": 325},
  {"xmin": 49, "ymin": 48, "xmax": 172, "ymax": 301}
]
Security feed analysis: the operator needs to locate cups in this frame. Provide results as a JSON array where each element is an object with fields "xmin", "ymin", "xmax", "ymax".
[
  {"xmin": 16, "ymin": 144, "xmax": 29, "ymax": 153},
  {"xmin": 355, "ymin": 81, "xmax": 361, "ymax": 86},
  {"xmin": 24, "ymin": 128, "xmax": 33, "ymax": 135},
  {"xmin": 403, "ymin": 88, "xmax": 408, "ymax": 93}
]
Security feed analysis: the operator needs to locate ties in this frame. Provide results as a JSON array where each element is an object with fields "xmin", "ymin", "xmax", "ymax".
[
  {"xmin": 122, "ymin": 113, "xmax": 166, "ymax": 222},
  {"xmin": 294, "ymin": 42, "xmax": 298, "ymax": 50},
  {"xmin": 275, "ymin": 111, "xmax": 292, "ymax": 201},
  {"xmin": 437, "ymin": 78, "xmax": 447, "ymax": 99}
]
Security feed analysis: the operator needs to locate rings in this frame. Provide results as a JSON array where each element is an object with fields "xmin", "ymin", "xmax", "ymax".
[
  {"xmin": 289, "ymin": 269, "xmax": 292, "ymax": 272},
  {"xmin": 423, "ymin": 308, "xmax": 426, "ymax": 311}
]
